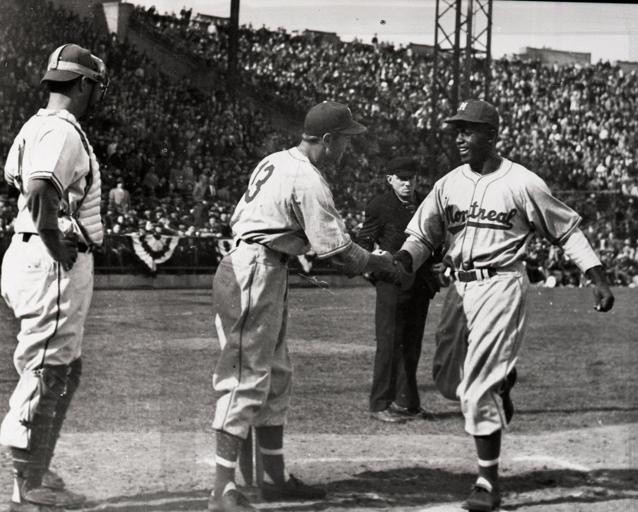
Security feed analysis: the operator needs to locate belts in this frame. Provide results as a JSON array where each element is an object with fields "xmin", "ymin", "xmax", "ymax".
[
  {"xmin": 21, "ymin": 233, "xmax": 88, "ymax": 255},
  {"xmin": 450, "ymin": 267, "xmax": 499, "ymax": 283}
]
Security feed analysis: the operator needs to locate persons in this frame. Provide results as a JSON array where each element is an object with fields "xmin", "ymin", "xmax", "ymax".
[
  {"xmin": 392, "ymin": 100, "xmax": 616, "ymax": 511},
  {"xmin": 359, "ymin": 156, "xmax": 441, "ymax": 423},
  {"xmin": 1, "ymin": 1, "xmax": 637, "ymax": 287},
  {"xmin": 207, "ymin": 100, "xmax": 398, "ymax": 512},
  {"xmin": 0, "ymin": 43, "xmax": 108, "ymax": 507}
]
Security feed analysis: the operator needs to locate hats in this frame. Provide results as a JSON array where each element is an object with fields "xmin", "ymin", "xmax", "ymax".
[
  {"xmin": 303, "ymin": 100, "xmax": 369, "ymax": 139},
  {"xmin": 444, "ymin": 100, "xmax": 500, "ymax": 132},
  {"xmin": 387, "ymin": 157, "xmax": 419, "ymax": 179}
]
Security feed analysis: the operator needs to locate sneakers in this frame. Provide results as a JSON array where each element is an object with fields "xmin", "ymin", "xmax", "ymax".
[
  {"xmin": 501, "ymin": 367, "xmax": 518, "ymax": 425},
  {"xmin": 461, "ymin": 483, "xmax": 502, "ymax": 512},
  {"xmin": 207, "ymin": 488, "xmax": 258, "ymax": 512},
  {"xmin": 12, "ymin": 469, "xmax": 88, "ymax": 512},
  {"xmin": 262, "ymin": 474, "xmax": 327, "ymax": 502},
  {"xmin": 369, "ymin": 403, "xmax": 436, "ymax": 423}
]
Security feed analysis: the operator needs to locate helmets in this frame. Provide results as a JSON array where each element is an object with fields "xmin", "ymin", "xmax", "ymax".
[{"xmin": 39, "ymin": 43, "xmax": 111, "ymax": 113}]
